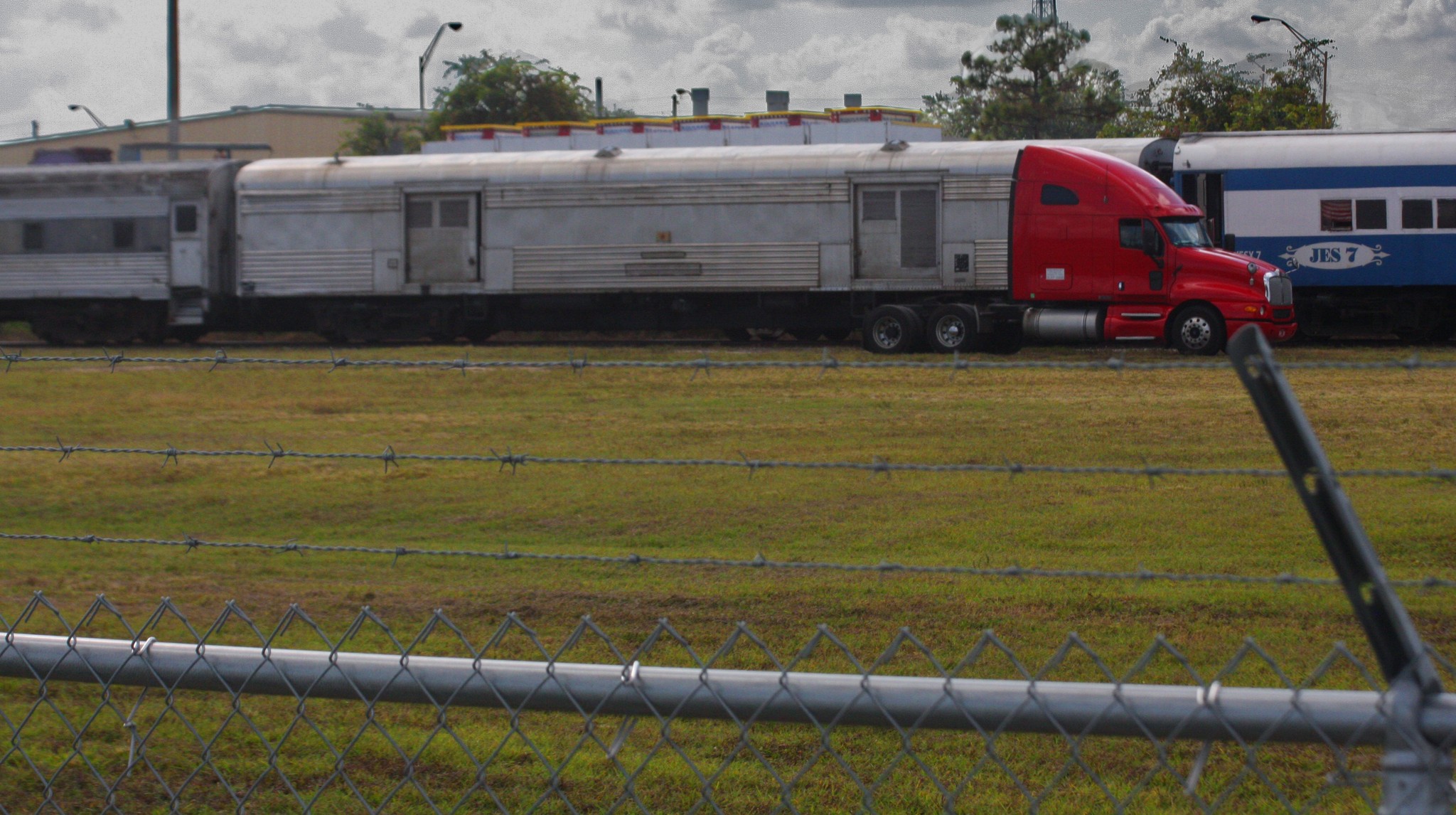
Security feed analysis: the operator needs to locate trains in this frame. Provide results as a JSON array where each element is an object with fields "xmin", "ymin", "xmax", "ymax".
[
  {"xmin": 1057, "ymin": 128, "xmax": 1456, "ymax": 347},
  {"xmin": 0, "ymin": 145, "xmax": 1297, "ymax": 356}
]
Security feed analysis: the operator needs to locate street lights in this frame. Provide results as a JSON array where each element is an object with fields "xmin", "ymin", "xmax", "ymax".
[
  {"xmin": 419, "ymin": 21, "xmax": 462, "ymax": 108},
  {"xmin": 1249, "ymin": 14, "xmax": 1328, "ymax": 129}
]
